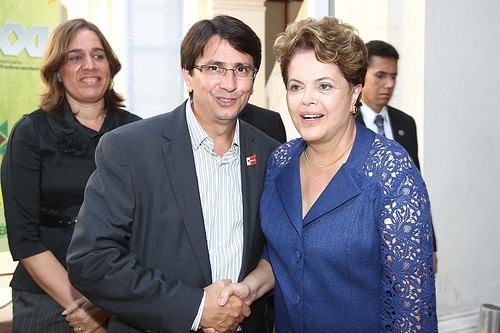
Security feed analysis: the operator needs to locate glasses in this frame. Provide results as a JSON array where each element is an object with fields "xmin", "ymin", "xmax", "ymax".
[{"xmin": 192, "ymin": 65, "xmax": 258, "ymax": 80}]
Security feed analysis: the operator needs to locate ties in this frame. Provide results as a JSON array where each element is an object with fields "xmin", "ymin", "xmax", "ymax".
[{"xmin": 375, "ymin": 114, "xmax": 388, "ymax": 136}]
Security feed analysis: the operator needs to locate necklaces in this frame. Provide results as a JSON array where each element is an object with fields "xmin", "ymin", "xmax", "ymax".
[{"xmin": 304, "ymin": 143, "xmax": 353, "ymax": 167}]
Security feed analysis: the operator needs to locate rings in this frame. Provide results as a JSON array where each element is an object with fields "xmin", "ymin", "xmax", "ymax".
[{"xmin": 78, "ymin": 327, "xmax": 82, "ymax": 332}]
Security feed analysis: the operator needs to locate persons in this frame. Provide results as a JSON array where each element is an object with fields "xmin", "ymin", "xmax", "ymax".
[
  {"xmin": 67, "ymin": 16, "xmax": 282, "ymax": 333},
  {"xmin": 353, "ymin": 40, "xmax": 440, "ymax": 273},
  {"xmin": 0, "ymin": 19, "xmax": 143, "ymax": 333},
  {"xmin": 239, "ymin": 103, "xmax": 287, "ymax": 144},
  {"xmin": 202, "ymin": 15, "xmax": 439, "ymax": 333}
]
